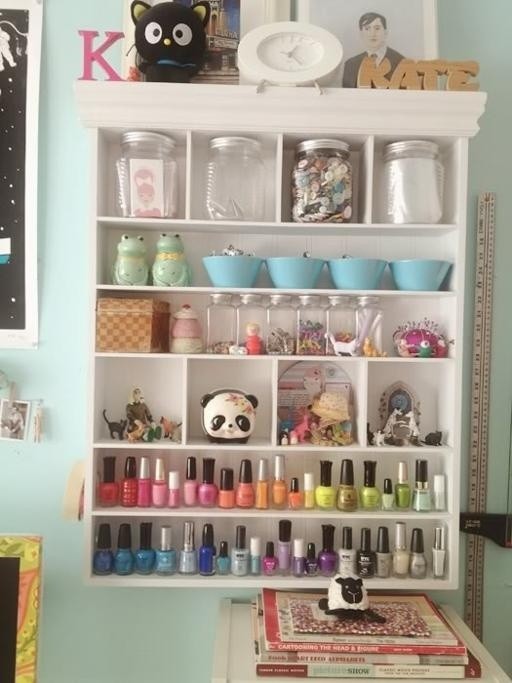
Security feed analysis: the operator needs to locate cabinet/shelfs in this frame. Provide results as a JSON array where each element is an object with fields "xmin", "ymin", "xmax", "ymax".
[{"xmin": 73, "ymin": 77, "xmax": 487, "ymax": 596}]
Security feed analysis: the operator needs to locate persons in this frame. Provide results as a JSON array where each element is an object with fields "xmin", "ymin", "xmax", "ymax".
[
  {"xmin": 340, "ymin": 12, "xmax": 412, "ymax": 88},
  {"xmin": 0, "ymin": 404, "xmax": 23, "ymax": 434}
]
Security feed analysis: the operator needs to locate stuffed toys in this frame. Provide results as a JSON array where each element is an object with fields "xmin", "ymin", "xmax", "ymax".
[
  {"xmin": 131, "ymin": 0, "xmax": 214, "ymax": 81},
  {"xmin": 316, "ymin": 571, "xmax": 386, "ymax": 626}
]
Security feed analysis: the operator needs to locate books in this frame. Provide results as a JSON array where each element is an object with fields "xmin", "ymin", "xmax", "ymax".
[{"xmin": 250, "ymin": 588, "xmax": 469, "ymax": 679}]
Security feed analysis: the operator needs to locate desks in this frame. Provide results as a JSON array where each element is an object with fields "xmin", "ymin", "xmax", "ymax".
[{"xmin": 212, "ymin": 596, "xmax": 512, "ymax": 683}]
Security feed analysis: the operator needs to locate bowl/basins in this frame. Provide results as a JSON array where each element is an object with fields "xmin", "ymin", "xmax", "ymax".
[{"xmin": 204, "ymin": 255, "xmax": 451, "ymax": 293}]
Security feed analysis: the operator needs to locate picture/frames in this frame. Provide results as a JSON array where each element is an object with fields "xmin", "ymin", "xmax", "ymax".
[{"xmin": 295, "ymin": 0, "xmax": 438, "ymax": 90}]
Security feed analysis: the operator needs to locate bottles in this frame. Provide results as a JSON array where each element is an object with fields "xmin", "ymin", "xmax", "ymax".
[
  {"xmin": 197, "ymin": 134, "xmax": 270, "ymax": 221},
  {"xmin": 205, "ymin": 294, "xmax": 385, "ymax": 355},
  {"xmin": 286, "ymin": 137, "xmax": 357, "ymax": 222},
  {"xmin": 99, "ymin": 130, "xmax": 181, "ymax": 221},
  {"xmin": 377, "ymin": 138, "xmax": 452, "ymax": 223}
]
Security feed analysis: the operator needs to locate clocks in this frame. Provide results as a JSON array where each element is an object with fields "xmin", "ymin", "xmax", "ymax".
[{"xmin": 236, "ymin": 21, "xmax": 344, "ymax": 94}]
